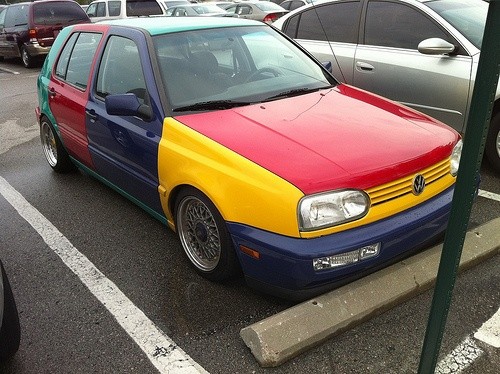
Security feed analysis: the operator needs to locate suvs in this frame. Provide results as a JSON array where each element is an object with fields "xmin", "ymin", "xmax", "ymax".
[
  {"xmin": 0, "ymin": 0, "xmax": 93, "ymax": 68},
  {"xmin": 85, "ymin": 0, "xmax": 168, "ymax": 24}
]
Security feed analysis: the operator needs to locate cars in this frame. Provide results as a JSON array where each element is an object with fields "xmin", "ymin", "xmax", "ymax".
[
  {"xmin": 223, "ymin": 1, "xmax": 291, "ymax": 25},
  {"xmin": 166, "ymin": 4, "xmax": 239, "ymax": 17},
  {"xmin": 35, "ymin": 17, "xmax": 463, "ymax": 303},
  {"xmin": 280, "ymin": 0, "xmax": 315, "ymax": 11},
  {"xmin": 271, "ymin": 0, "xmax": 500, "ymax": 178}
]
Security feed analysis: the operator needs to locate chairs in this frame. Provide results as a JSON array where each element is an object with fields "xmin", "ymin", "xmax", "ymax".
[{"xmin": 189, "ymin": 49, "xmax": 231, "ymax": 96}]
